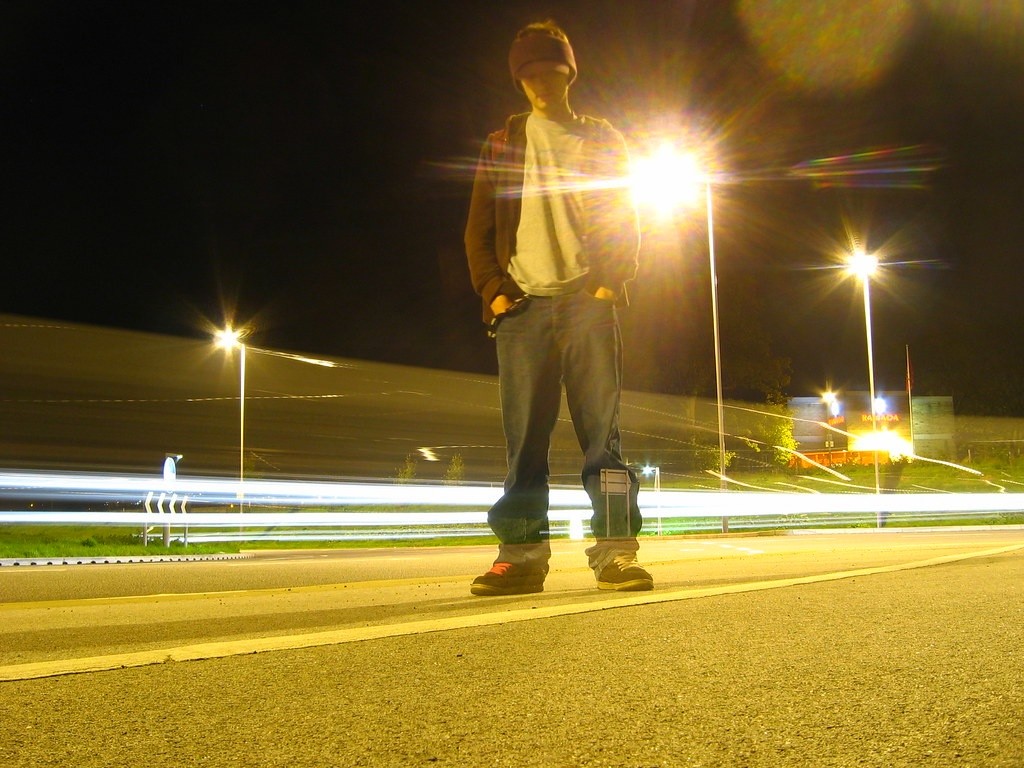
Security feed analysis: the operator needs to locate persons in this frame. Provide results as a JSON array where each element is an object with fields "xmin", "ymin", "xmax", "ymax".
[{"xmin": 461, "ymin": 22, "xmax": 656, "ymax": 599}]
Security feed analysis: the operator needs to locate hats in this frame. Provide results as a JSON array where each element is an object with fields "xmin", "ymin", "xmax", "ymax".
[{"xmin": 508, "ymin": 22, "xmax": 576, "ymax": 91}]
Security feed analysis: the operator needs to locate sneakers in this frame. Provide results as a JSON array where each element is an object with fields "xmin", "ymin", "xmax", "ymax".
[
  {"xmin": 471, "ymin": 562, "xmax": 550, "ymax": 595},
  {"xmin": 598, "ymin": 554, "xmax": 653, "ymax": 590}
]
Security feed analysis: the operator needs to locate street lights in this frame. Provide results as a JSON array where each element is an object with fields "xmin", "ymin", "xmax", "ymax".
[
  {"xmin": 848, "ymin": 253, "xmax": 881, "ymax": 528},
  {"xmin": 642, "ymin": 464, "xmax": 663, "ymax": 535},
  {"xmin": 220, "ymin": 332, "xmax": 245, "ymax": 482},
  {"xmin": 643, "ymin": 151, "xmax": 729, "ymax": 535}
]
